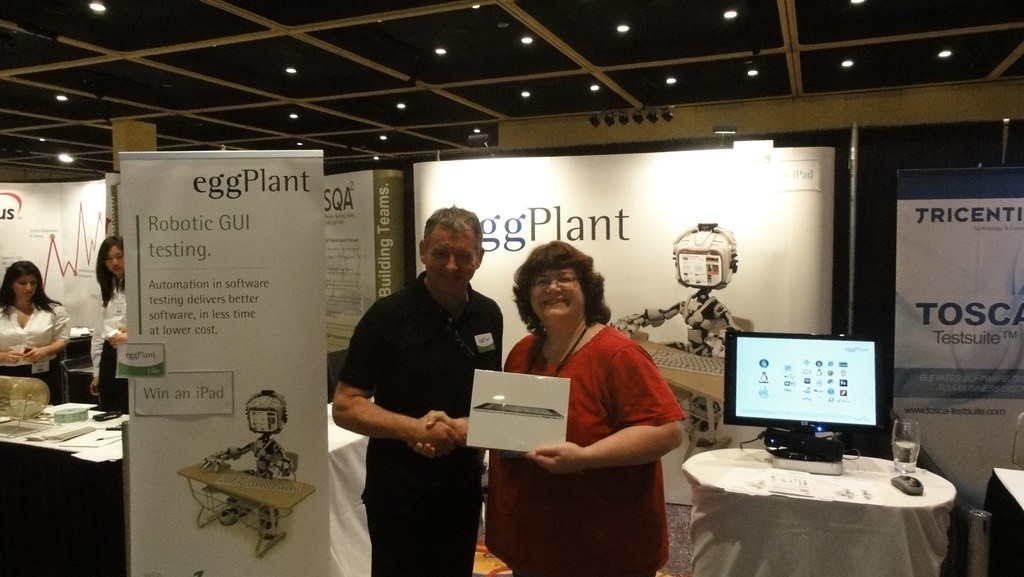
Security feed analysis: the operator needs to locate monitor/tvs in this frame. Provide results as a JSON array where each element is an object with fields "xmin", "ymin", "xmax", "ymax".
[{"xmin": 723, "ymin": 329, "xmax": 879, "ymax": 461}]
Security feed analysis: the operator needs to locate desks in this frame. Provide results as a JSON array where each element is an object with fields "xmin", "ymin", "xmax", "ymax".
[
  {"xmin": 327, "ymin": 401, "xmax": 373, "ymax": 577},
  {"xmin": 177, "ymin": 460, "xmax": 317, "ymax": 560},
  {"xmin": 680, "ymin": 449, "xmax": 959, "ymax": 577},
  {"xmin": 0, "ymin": 335, "xmax": 129, "ymax": 575},
  {"xmin": 984, "ymin": 468, "xmax": 1024, "ymax": 577}
]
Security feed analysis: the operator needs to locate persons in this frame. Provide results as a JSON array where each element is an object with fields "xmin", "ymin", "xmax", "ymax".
[
  {"xmin": 332, "ymin": 206, "xmax": 504, "ymax": 577},
  {"xmin": 0, "ymin": 260, "xmax": 71, "ymax": 404},
  {"xmin": 90, "ymin": 236, "xmax": 129, "ymax": 415},
  {"xmin": 409, "ymin": 240, "xmax": 686, "ymax": 577}
]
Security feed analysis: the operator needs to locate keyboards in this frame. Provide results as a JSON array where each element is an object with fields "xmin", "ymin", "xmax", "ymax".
[{"xmin": 645, "ymin": 347, "xmax": 724, "ymax": 377}]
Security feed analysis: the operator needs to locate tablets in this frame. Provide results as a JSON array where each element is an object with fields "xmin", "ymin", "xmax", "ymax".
[{"xmin": 473, "ymin": 403, "xmax": 564, "ymax": 420}]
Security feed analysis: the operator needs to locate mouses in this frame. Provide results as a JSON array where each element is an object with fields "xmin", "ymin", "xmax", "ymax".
[{"xmin": 891, "ymin": 475, "xmax": 923, "ymax": 495}]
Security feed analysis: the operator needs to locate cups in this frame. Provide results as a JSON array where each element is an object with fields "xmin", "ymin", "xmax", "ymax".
[{"xmin": 891, "ymin": 418, "xmax": 920, "ymax": 473}]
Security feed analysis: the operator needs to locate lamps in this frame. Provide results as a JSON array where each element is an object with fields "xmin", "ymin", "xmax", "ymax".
[
  {"xmin": 468, "ymin": 133, "xmax": 489, "ymax": 148},
  {"xmin": 712, "ymin": 123, "xmax": 737, "ymax": 143},
  {"xmin": 588, "ymin": 106, "xmax": 673, "ymax": 127}
]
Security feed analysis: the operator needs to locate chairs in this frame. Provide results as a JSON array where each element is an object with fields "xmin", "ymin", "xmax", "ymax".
[{"xmin": 276, "ymin": 451, "xmax": 300, "ymax": 518}]
